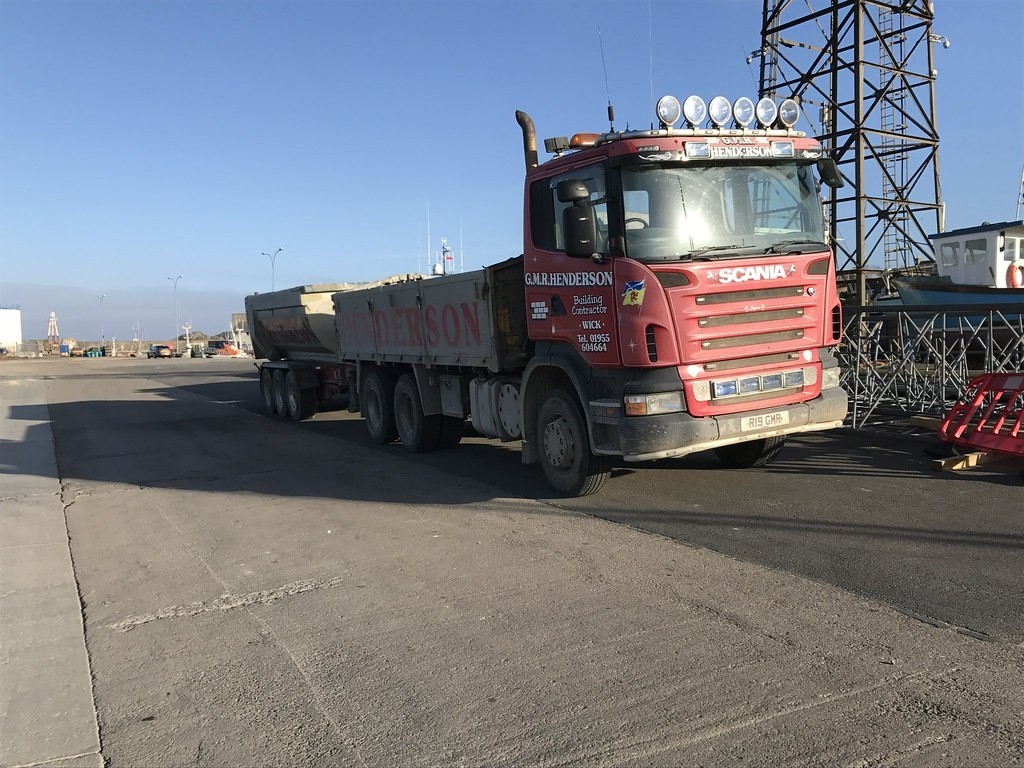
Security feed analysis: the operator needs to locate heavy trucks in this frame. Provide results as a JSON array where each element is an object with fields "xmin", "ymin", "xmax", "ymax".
[{"xmin": 243, "ymin": 97, "xmax": 849, "ymax": 498}]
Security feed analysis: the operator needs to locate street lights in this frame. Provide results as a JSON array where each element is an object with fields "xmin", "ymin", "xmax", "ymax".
[
  {"xmin": 98, "ymin": 294, "xmax": 106, "ymax": 346},
  {"xmin": 259, "ymin": 246, "xmax": 283, "ymax": 293},
  {"xmin": 168, "ymin": 274, "xmax": 183, "ymax": 354}
]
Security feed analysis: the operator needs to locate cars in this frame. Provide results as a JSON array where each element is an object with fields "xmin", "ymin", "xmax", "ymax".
[{"xmin": 147, "ymin": 344, "xmax": 173, "ymax": 359}]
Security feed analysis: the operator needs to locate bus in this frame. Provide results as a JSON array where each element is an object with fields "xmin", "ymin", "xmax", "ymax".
[{"xmin": 205, "ymin": 340, "xmax": 235, "ymax": 354}]
